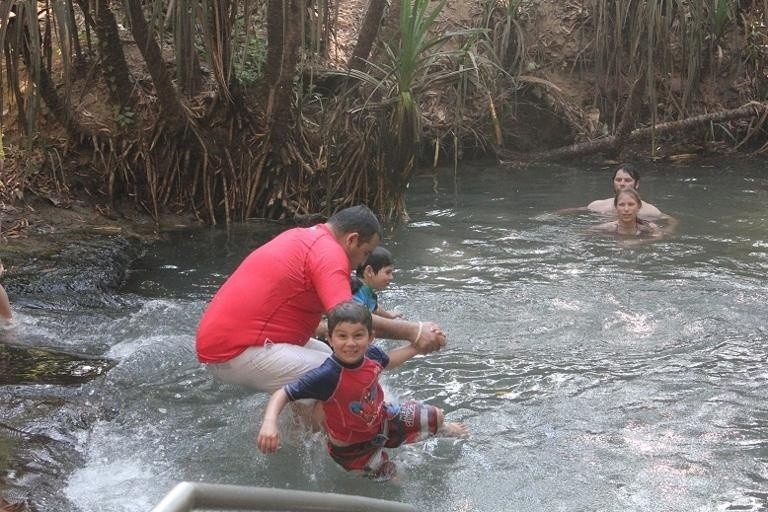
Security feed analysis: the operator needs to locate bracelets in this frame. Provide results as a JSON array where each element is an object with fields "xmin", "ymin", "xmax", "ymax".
[{"xmin": 412, "ymin": 320, "xmax": 422, "ymax": 346}]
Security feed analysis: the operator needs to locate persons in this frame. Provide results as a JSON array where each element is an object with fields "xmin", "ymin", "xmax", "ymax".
[
  {"xmin": 256, "ymin": 300, "xmax": 465, "ymax": 488},
  {"xmin": 558, "ymin": 163, "xmax": 678, "ymax": 237},
  {"xmin": 588, "ymin": 187, "xmax": 663, "ymax": 247},
  {"xmin": 307, "ymin": 246, "xmax": 404, "ymax": 320},
  {"xmin": 0, "ymin": 260, "xmax": 12, "ymax": 320},
  {"xmin": 197, "ymin": 204, "xmax": 446, "ymax": 433}
]
